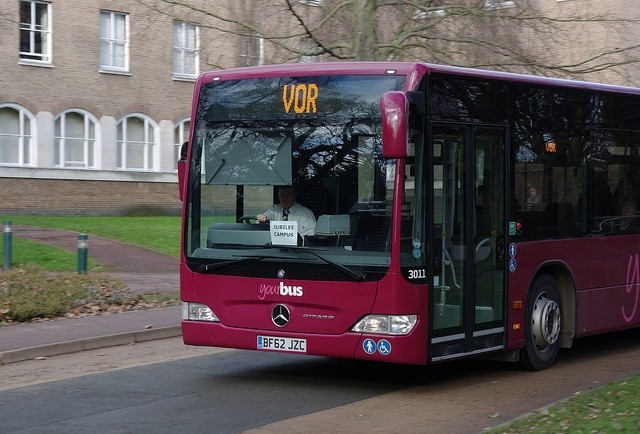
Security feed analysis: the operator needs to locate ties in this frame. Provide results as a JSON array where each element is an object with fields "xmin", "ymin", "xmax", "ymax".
[{"xmin": 282, "ymin": 210, "xmax": 290, "ymax": 221}]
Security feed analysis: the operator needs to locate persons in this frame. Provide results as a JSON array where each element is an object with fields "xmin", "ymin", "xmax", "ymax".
[
  {"xmin": 527, "ymin": 187, "xmax": 543, "ymax": 210},
  {"xmin": 257, "ymin": 186, "xmax": 316, "ymax": 247}
]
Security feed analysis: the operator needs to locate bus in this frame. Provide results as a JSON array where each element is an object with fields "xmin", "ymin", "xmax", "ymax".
[{"xmin": 178, "ymin": 62, "xmax": 639, "ymax": 371}]
